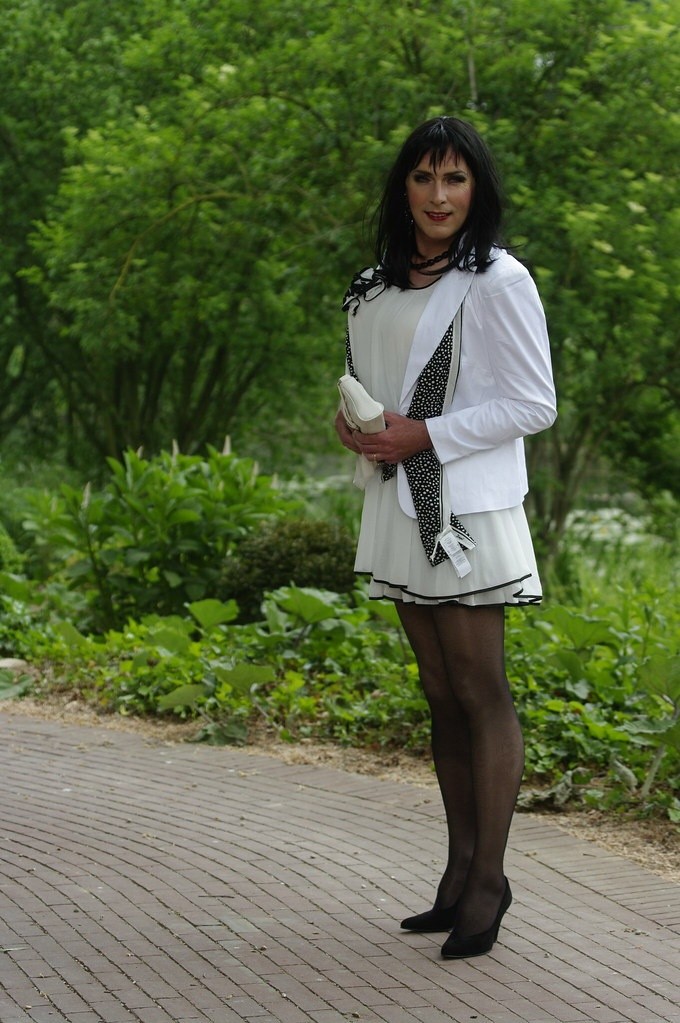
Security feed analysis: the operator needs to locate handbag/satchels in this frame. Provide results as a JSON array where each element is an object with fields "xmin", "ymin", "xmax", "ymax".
[{"xmin": 337, "ymin": 374, "xmax": 386, "ymax": 434}]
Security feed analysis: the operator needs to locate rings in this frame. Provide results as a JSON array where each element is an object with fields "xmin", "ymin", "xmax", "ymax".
[{"xmin": 374, "ymin": 453, "xmax": 376, "ymax": 462}]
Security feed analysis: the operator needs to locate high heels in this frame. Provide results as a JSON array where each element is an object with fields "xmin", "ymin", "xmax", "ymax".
[
  {"xmin": 401, "ymin": 870, "xmax": 470, "ymax": 933},
  {"xmin": 441, "ymin": 875, "xmax": 513, "ymax": 959}
]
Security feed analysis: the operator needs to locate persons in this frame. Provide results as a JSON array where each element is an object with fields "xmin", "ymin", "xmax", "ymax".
[{"xmin": 335, "ymin": 115, "xmax": 558, "ymax": 957}]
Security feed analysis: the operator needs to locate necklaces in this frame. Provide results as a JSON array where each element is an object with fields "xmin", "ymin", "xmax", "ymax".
[{"xmin": 409, "ymin": 250, "xmax": 448, "ymax": 270}]
